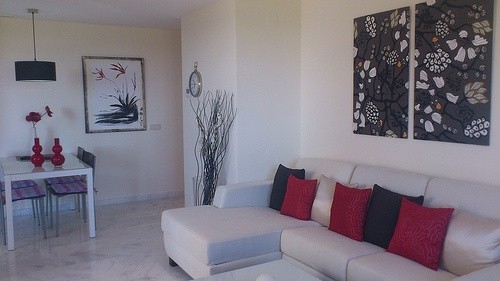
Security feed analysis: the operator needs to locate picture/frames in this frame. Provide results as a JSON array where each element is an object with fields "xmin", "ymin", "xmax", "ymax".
[{"xmin": 81, "ymin": 56, "xmax": 146, "ymax": 134}]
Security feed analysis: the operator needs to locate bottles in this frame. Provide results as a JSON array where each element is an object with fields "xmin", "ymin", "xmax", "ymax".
[{"xmin": 51, "ymin": 138, "xmax": 65, "ymax": 168}]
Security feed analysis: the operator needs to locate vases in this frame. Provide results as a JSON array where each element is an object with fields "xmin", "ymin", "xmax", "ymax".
[
  {"xmin": 50, "ymin": 138, "xmax": 65, "ymax": 167},
  {"xmin": 192, "ymin": 176, "xmax": 220, "ymax": 206},
  {"xmin": 31, "ymin": 137, "xmax": 44, "ymax": 168}
]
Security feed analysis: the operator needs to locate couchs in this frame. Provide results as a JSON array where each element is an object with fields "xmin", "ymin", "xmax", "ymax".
[{"xmin": 160, "ymin": 157, "xmax": 500, "ymax": 281}]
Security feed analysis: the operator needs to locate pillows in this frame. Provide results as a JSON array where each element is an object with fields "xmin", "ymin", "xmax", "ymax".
[
  {"xmin": 439, "ymin": 203, "xmax": 500, "ymax": 276},
  {"xmin": 280, "ymin": 174, "xmax": 318, "ymax": 221},
  {"xmin": 327, "ymin": 181, "xmax": 372, "ymax": 243},
  {"xmin": 309, "ymin": 174, "xmax": 359, "ymax": 228},
  {"xmin": 385, "ymin": 194, "xmax": 455, "ymax": 272},
  {"xmin": 268, "ymin": 164, "xmax": 305, "ymax": 210},
  {"xmin": 363, "ymin": 183, "xmax": 425, "ymax": 249}
]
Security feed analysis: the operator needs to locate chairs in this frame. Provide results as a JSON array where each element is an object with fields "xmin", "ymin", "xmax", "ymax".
[{"xmin": 0, "ymin": 146, "xmax": 97, "ymax": 245}]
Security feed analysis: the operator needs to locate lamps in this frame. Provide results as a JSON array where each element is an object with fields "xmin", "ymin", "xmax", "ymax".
[{"xmin": 14, "ymin": 9, "xmax": 57, "ymax": 83}]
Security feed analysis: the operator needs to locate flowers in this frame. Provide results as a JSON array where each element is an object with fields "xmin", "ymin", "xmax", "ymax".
[{"xmin": 25, "ymin": 105, "xmax": 53, "ymax": 137}]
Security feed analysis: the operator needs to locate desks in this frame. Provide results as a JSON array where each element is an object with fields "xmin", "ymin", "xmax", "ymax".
[{"xmin": 0, "ymin": 152, "xmax": 95, "ymax": 251}]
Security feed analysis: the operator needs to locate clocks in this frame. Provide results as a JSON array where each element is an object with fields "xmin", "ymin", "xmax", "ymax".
[{"xmin": 189, "ymin": 62, "xmax": 202, "ymax": 97}]
